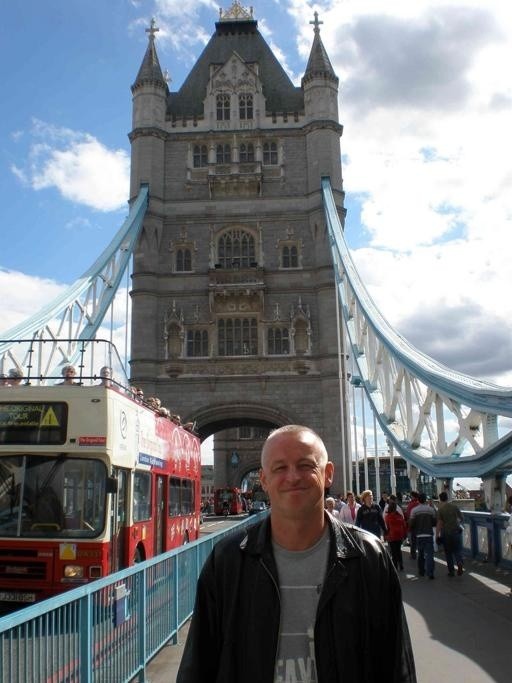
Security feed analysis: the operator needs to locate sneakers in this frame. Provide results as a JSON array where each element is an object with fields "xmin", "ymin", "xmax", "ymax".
[
  {"xmin": 399, "ymin": 562, "xmax": 404, "ymax": 571},
  {"xmin": 448, "ymin": 560, "xmax": 463, "ymax": 577}
]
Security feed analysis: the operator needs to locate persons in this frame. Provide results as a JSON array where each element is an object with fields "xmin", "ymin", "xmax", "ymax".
[
  {"xmin": 126, "ymin": 386, "xmax": 194, "ymax": 433},
  {"xmin": 96, "ymin": 366, "xmax": 118, "ymax": 393},
  {"xmin": 55, "ymin": 367, "xmax": 80, "ymax": 388},
  {"xmin": 16, "ymin": 484, "xmax": 66, "ymax": 531},
  {"xmin": 1, "ymin": 374, "xmax": 8, "ymax": 386},
  {"xmin": 7, "ymin": 368, "xmax": 24, "ymax": 387},
  {"xmin": 175, "ymin": 425, "xmax": 418, "ymax": 682},
  {"xmin": 168, "ymin": 495, "xmax": 215, "ymax": 517},
  {"xmin": 324, "ymin": 489, "xmax": 490, "ymax": 579},
  {"xmin": 223, "ymin": 500, "xmax": 229, "ymax": 510}
]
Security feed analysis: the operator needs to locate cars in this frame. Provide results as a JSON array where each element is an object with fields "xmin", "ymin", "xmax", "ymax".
[{"xmin": 248, "ymin": 500, "xmax": 267, "ymax": 515}]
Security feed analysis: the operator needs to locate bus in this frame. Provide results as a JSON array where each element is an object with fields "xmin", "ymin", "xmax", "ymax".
[
  {"xmin": 1, "ymin": 335, "xmax": 204, "ymax": 614},
  {"xmin": 214, "ymin": 487, "xmax": 244, "ymax": 516}
]
